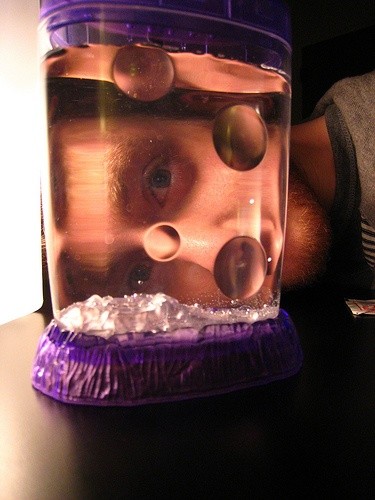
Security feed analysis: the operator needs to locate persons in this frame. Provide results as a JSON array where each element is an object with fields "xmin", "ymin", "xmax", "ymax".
[{"xmin": 48, "ymin": 72, "xmax": 374, "ymax": 308}]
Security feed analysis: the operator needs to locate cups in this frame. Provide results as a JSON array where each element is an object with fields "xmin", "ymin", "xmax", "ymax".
[{"xmin": 32, "ymin": 0, "xmax": 366, "ymax": 408}]
